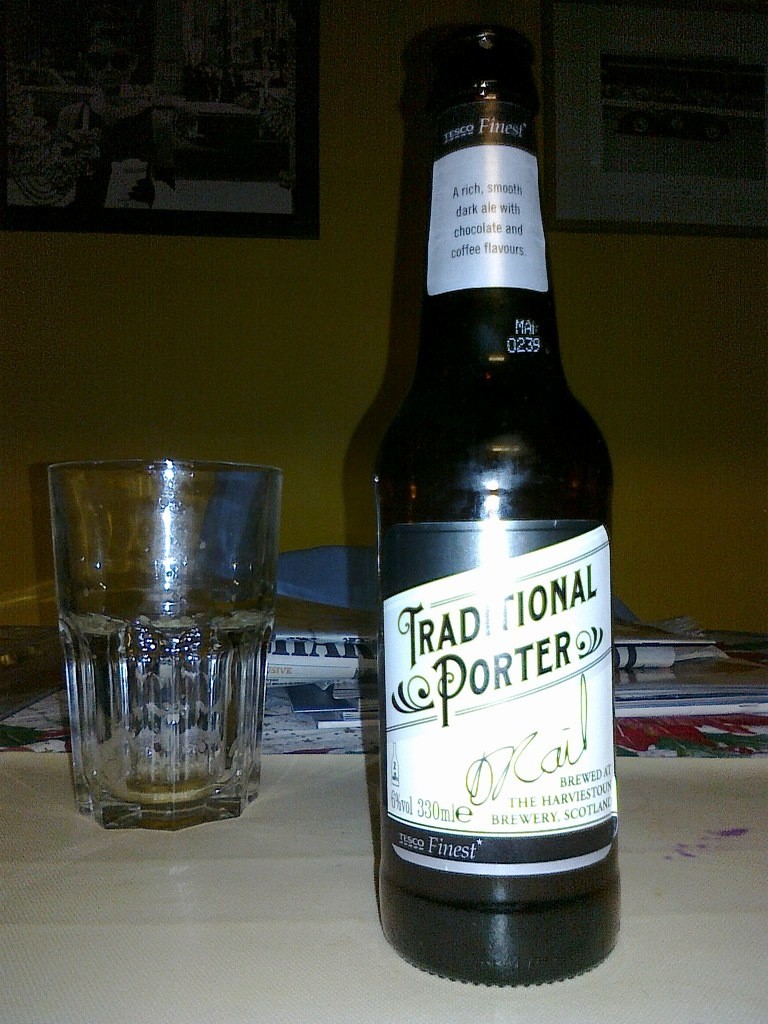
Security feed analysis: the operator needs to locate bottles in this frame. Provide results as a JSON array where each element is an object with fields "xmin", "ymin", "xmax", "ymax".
[{"xmin": 378, "ymin": 28, "xmax": 623, "ymax": 989}]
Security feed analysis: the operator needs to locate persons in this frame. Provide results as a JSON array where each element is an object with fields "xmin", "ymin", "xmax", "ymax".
[{"xmin": 52, "ymin": 4, "xmax": 165, "ymax": 207}]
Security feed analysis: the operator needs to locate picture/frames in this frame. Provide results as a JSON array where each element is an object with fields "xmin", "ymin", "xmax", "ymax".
[
  {"xmin": 536, "ymin": 0, "xmax": 768, "ymax": 238},
  {"xmin": 0, "ymin": 0, "xmax": 321, "ymax": 242}
]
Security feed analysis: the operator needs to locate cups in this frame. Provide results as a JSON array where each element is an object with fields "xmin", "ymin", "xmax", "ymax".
[{"xmin": 48, "ymin": 459, "xmax": 284, "ymax": 831}]
